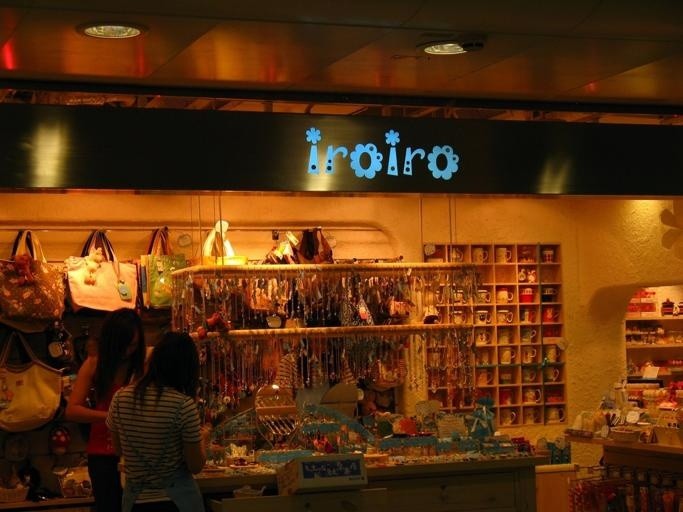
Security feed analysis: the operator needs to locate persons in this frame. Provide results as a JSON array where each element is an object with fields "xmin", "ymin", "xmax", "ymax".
[
  {"xmin": 105, "ymin": 330, "xmax": 208, "ymax": 512},
  {"xmin": 64, "ymin": 307, "xmax": 155, "ymax": 512}
]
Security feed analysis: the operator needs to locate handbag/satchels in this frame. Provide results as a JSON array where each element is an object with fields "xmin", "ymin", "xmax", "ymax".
[
  {"xmin": 0, "ymin": 230, "xmax": 187, "ymax": 322},
  {"xmin": 190, "ymin": 229, "xmax": 248, "ymax": 265},
  {"xmin": 0, "ymin": 333, "xmax": 64, "ymax": 432}
]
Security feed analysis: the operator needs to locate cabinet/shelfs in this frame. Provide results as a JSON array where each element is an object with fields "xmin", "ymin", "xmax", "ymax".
[{"xmin": 622, "ymin": 309, "xmax": 682, "ymax": 414}]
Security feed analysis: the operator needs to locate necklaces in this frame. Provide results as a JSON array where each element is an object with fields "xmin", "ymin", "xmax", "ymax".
[{"xmin": 170, "ymin": 267, "xmax": 478, "ymax": 419}]
